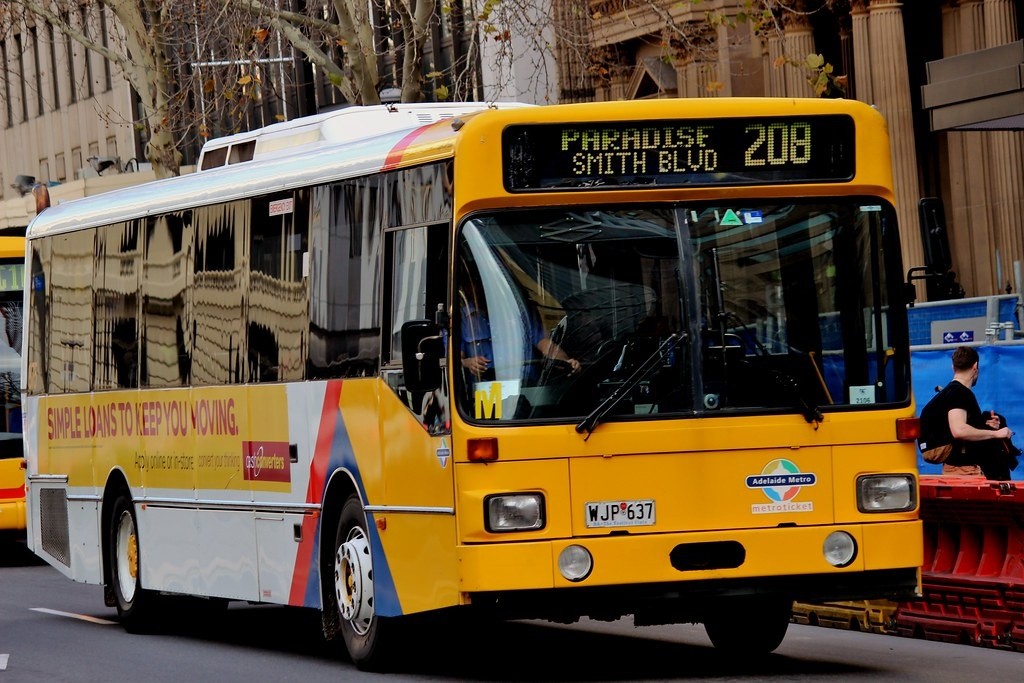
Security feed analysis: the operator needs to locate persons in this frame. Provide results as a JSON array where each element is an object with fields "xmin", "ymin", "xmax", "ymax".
[
  {"xmin": 939, "ymin": 346, "xmax": 1012, "ymax": 481},
  {"xmin": 441, "ymin": 276, "xmax": 583, "ymax": 383}
]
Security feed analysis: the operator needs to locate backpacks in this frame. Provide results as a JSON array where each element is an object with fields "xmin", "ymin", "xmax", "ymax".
[{"xmin": 918, "ymin": 385, "xmax": 971, "ymax": 464}]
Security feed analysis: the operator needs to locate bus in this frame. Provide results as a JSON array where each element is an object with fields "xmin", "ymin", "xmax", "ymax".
[
  {"xmin": 0, "ymin": 235, "xmax": 27, "ymax": 530},
  {"xmin": 20, "ymin": 88, "xmax": 966, "ymax": 673}
]
